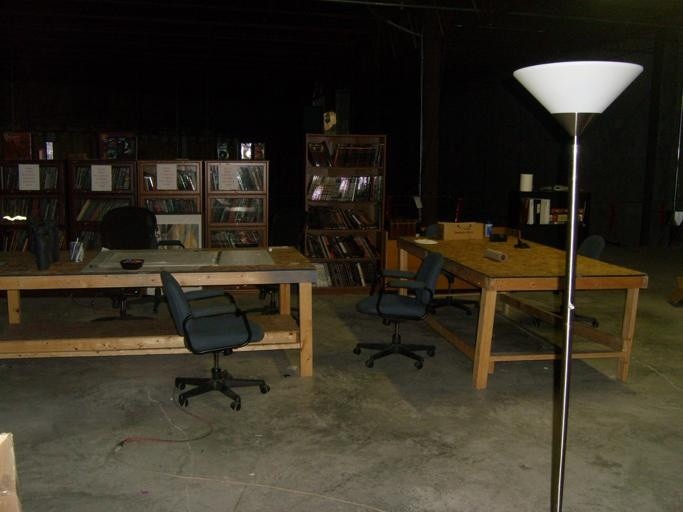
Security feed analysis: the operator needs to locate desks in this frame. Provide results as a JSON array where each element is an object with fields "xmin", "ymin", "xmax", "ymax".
[
  {"xmin": 0, "ymin": 246, "xmax": 318, "ymax": 378},
  {"xmin": 398, "ymin": 236, "xmax": 649, "ymax": 390}
]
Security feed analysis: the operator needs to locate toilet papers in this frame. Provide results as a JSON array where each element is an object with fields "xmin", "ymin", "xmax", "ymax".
[{"xmin": 483, "ymin": 248, "xmax": 508, "ymax": 262}]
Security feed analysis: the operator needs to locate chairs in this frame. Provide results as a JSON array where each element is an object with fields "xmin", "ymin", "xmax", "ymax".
[
  {"xmin": 160, "ymin": 271, "xmax": 271, "ymax": 411},
  {"xmin": 353, "ymin": 253, "xmax": 443, "ymax": 369},
  {"xmin": 527, "ymin": 236, "xmax": 606, "ymax": 328},
  {"xmin": 97, "ymin": 205, "xmax": 184, "ymax": 324},
  {"xmin": 425, "ymin": 224, "xmax": 478, "ymax": 315}
]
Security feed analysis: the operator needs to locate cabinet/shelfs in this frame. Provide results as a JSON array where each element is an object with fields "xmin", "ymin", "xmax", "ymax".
[
  {"xmin": 2, "ymin": 131, "xmax": 135, "ymax": 248},
  {"xmin": 137, "ymin": 131, "xmax": 270, "ymax": 295},
  {"xmin": 510, "ymin": 188, "xmax": 590, "ymax": 250},
  {"xmin": 305, "ymin": 133, "xmax": 392, "ymax": 295}
]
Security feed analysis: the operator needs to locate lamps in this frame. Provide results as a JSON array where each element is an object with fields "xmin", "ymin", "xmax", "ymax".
[{"xmin": 513, "ymin": 60, "xmax": 643, "ymax": 512}]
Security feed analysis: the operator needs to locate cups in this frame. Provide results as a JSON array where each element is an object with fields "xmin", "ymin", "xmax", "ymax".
[{"xmin": 68, "ymin": 241, "xmax": 85, "ymax": 264}]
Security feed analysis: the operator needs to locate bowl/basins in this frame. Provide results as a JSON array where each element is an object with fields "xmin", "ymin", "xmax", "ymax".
[{"xmin": 120, "ymin": 258, "xmax": 144, "ymax": 270}]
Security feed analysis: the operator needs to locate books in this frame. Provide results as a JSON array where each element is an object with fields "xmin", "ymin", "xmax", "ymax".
[
  {"xmin": 0, "ymin": 132, "xmax": 262, "ymax": 247},
  {"xmin": 308, "ymin": 139, "xmax": 385, "ymax": 287},
  {"xmin": 522, "ymin": 198, "xmax": 584, "ymax": 225}
]
